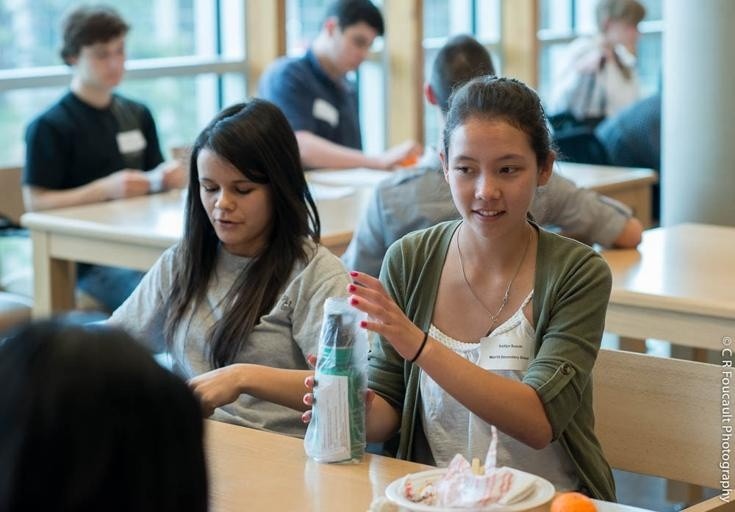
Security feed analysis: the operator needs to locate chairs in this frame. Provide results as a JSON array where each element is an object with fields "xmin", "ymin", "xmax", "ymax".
[
  {"xmin": 2, "ymin": 167, "xmax": 106, "ymax": 317},
  {"xmin": 575, "ymin": 346, "xmax": 735, "ymax": 512}
]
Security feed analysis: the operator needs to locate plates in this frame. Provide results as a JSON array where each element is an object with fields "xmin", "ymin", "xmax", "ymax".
[{"xmin": 384, "ymin": 467, "xmax": 556, "ymax": 511}]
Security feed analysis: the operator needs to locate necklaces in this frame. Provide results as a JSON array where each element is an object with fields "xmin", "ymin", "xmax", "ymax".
[{"xmin": 456, "ymin": 218, "xmax": 531, "ymax": 338}]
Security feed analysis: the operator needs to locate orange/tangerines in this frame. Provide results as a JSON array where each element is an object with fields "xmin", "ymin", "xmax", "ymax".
[{"xmin": 550, "ymin": 491, "xmax": 597, "ymax": 512}]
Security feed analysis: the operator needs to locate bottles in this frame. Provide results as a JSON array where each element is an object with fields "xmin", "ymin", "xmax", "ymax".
[{"xmin": 312, "ymin": 309, "xmax": 367, "ymax": 464}]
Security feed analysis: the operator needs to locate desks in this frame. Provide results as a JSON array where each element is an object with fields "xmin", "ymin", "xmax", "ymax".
[
  {"xmin": 595, "ymin": 220, "xmax": 734, "ymax": 364},
  {"xmin": 26, "ymin": 149, "xmax": 662, "ymax": 328},
  {"xmin": 202, "ymin": 418, "xmax": 562, "ymax": 511}
]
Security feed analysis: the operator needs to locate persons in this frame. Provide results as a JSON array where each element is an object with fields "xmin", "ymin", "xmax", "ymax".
[
  {"xmin": 302, "ymin": 77, "xmax": 615, "ymax": 501},
  {"xmin": 546, "ymin": 1, "xmax": 662, "ymax": 227},
  {"xmin": 256, "ymin": 0, "xmax": 420, "ymax": 171},
  {"xmin": 80, "ymin": 98, "xmax": 355, "ymax": 440},
  {"xmin": 1, "ymin": 317, "xmax": 210, "ymax": 511},
  {"xmin": 21, "ymin": 4, "xmax": 189, "ymax": 314},
  {"xmin": 335, "ymin": 33, "xmax": 643, "ymax": 297}
]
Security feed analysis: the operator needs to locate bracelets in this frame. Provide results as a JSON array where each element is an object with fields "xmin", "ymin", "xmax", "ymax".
[{"xmin": 410, "ymin": 332, "xmax": 428, "ymax": 364}]
